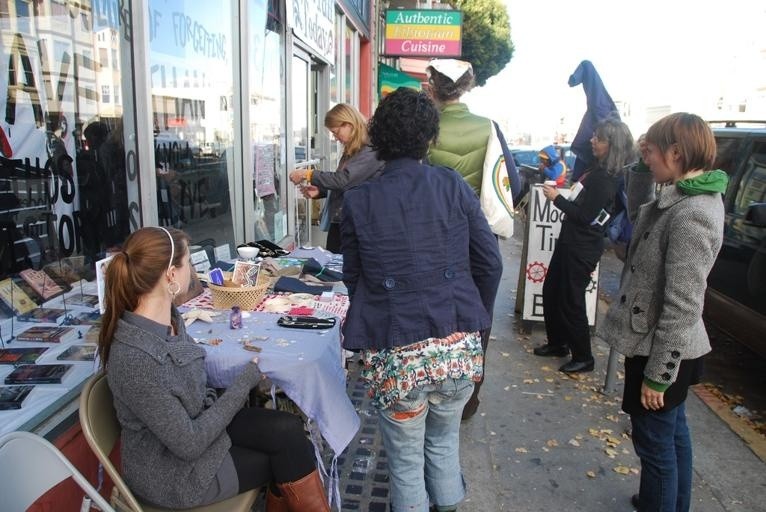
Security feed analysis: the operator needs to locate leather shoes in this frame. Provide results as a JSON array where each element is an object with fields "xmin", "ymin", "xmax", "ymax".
[
  {"xmin": 560, "ymin": 357, "xmax": 594, "ymax": 372},
  {"xmin": 535, "ymin": 345, "xmax": 569, "ymax": 356}
]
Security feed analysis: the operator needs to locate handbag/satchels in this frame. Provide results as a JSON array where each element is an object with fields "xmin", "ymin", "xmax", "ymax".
[
  {"xmin": 480, "ymin": 134, "xmax": 515, "ymax": 239},
  {"xmin": 319, "ymin": 190, "xmax": 331, "ymax": 231}
]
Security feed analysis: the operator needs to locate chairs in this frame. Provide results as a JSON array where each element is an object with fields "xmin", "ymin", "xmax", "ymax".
[
  {"xmin": 0, "ymin": 430, "xmax": 114, "ymax": 512},
  {"xmin": 78, "ymin": 368, "xmax": 263, "ymax": 512}
]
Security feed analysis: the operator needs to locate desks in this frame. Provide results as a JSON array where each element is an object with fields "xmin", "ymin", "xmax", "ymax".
[{"xmin": 175, "ymin": 247, "xmax": 361, "ymax": 512}]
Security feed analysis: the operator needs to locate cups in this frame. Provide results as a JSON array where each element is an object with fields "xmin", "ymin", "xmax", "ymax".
[{"xmin": 544, "ymin": 180, "xmax": 557, "ymax": 187}]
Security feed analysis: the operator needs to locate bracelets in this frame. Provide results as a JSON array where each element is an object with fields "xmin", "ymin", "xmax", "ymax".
[{"xmin": 307, "ymin": 170, "xmax": 313, "ymax": 182}]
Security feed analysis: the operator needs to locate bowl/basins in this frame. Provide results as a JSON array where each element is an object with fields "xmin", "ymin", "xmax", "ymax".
[{"xmin": 237, "ymin": 246, "xmax": 260, "ymax": 260}]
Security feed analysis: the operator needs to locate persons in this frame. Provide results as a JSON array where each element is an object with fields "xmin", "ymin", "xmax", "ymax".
[
  {"xmin": 531, "ymin": 58, "xmax": 635, "ymax": 374},
  {"xmin": 596, "ymin": 110, "xmax": 730, "ymax": 512},
  {"xmin": 97, "ymin": 222, "xmax": 334, "ymax": 512},
  {"xmin": 290, "ymin": 105, "xmax": 387, "ymax": 254},
  {"xmin": 49, "ymin": 115, "xmax": 75, "ymax": 180},
  {"xmin": 420, "ymin": 59, "xmax": 521, "ymax": 423},
  {"xmin": 329, "ymin": 86, "xmax": 502, "ymax": 512},
  {"xmin": 537, "ymin": 144, "xmax": 567, "ymax": 189},
  {"xmin": 75, "ymin": 120, "xmax": 130, "ymax": 252}
]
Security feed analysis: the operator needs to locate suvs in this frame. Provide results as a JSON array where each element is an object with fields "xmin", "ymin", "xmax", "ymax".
[{"xmin": 701, "ymin": 119, "xmax": 764, "ymax": 360}]
